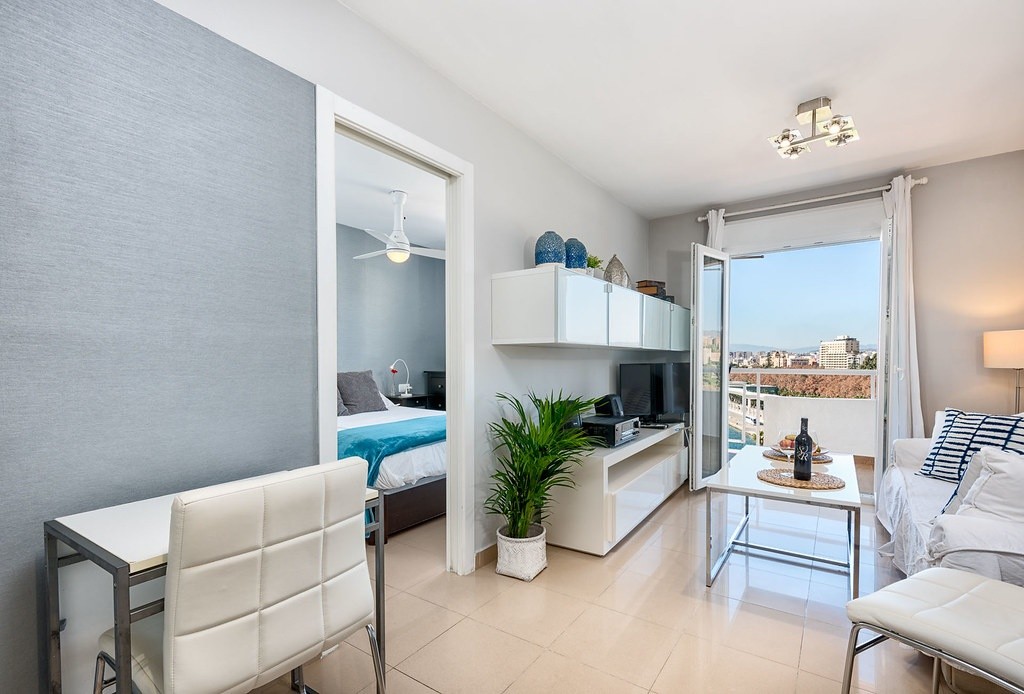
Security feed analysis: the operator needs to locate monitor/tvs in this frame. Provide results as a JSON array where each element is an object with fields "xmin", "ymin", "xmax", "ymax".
[{"xmin": 620, "ymin": 362, "xmax": 673, "ymax": 419}]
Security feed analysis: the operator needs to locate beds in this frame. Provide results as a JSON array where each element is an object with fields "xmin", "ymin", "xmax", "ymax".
[{"xmin": 336, "ymin": 389, "xmax": 446, "ymax": 546}]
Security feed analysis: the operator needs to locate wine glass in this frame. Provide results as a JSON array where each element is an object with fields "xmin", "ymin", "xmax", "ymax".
[
  {"xmin": 780, "ymin": 431, "xmax": 796, "ymax": 478},
  {"xmin": 806, "ymin": 428, "xmax": 818, "ymax": 476}
]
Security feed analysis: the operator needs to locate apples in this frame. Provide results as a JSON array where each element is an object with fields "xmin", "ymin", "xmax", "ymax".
[{"xmin": 780, "ymin": 439, "xmax": 796, "ymax": 449}]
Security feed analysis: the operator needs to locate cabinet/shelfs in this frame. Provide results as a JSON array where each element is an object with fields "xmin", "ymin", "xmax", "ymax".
[
  {"xmin": 422, "ymin": 370, "xmax": 445, "ymax": 410},
  {"xmin": 490, "ymin": 266, "xmax": 691, "ymax": 353},
  {"xmin": 540, "ymin": 424, "xmax": 689, "ymax": 555}
]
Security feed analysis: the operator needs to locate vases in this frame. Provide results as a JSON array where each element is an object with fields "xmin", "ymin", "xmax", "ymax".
[
  {"xmin": 604, "ymin": 253, "xmax": 629, "ymax": 287},
  {"xmin": 536, "ymin": 230, "xmax": 566, "ymax": 268},
  {"xmin": 564, "ymin": 238, "xmax": 587, "ymax": 274}
]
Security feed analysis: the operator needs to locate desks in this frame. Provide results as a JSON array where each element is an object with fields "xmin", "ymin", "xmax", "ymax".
[
  {"xmin": 706, "ymin": 442, "xmax": 859, "ymax": 615},
  {"xmin": 42, "ymin": 470, "xmax": 386, "ymax": 694}
]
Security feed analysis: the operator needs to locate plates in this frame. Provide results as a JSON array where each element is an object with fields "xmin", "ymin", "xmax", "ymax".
[{"xmin": 772, "ymin": 443, "xmax": 829, "ymax": 456}]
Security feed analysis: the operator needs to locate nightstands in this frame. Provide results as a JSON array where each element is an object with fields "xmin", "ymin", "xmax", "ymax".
[{"xmin": 384, "ymin": 392, "xmax": 428, "ymax": 407}]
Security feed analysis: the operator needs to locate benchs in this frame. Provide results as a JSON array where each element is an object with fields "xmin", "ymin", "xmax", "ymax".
[{"xmin": 839, "ymin": 566, "xmax": 1024, "ymax": 694}]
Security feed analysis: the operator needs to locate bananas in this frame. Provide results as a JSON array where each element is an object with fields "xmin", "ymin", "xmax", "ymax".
[{"xmin": 785, "ymin": 435, "xmax": 821, "ymax": 452}]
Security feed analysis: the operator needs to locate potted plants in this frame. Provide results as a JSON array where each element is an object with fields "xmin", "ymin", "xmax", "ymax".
[
  {"xmin": 585, "ymin": 254, "xmax": 604, "ymax": 277},
  {"xmin": 483, "ymin": 386, "xmax": 612, "ymax": 583}
]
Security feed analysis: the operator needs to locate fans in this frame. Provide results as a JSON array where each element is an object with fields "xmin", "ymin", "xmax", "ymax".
[{"xmin": 352, "ymin": 190, "xmax": 446, "ymax": 260}]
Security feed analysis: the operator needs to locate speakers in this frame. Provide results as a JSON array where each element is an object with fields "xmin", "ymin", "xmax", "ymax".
[{"xmin": 594, "ymin": 394, "xmax": 624, "ymax": 417}]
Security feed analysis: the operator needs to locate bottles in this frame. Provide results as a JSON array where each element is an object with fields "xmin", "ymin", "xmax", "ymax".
[
  {"xmin": 565, "ymin": 237, "xmax": 587, "ymax": 274},
  {"xmin": 535, "ymin": 231, "xmax": 567, "ymax": 267},
  {"xmin": 390, "ymin": 382, "xmax": 396, "ymax": 396},
  {"xmin": 604, "ymin": 255, "xmax": 630, "ymax": 287},
  {"xmin": 795, "ymin": 418, "xmax": 812, "ymax": 480}
]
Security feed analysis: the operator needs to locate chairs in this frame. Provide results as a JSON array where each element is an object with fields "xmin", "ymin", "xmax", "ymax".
[{"xmin": 92, "ymin": 456, "xmax": 386, "ymax": 694}]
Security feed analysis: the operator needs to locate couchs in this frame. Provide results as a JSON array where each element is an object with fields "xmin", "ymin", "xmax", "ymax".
[{"xmin": 876, "ymin": 438, "xmax": 1023, "ymax": 589}]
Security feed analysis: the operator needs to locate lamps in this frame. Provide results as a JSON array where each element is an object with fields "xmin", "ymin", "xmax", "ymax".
[
  {"xmin": 982, "ymin": 330, "xmax": 1024, "ymax": 415},
  {"xmin": 385, "ymin": 249, "xmax": 410, "ymax": 263},
  {"xmin": 766, "ymin": 96, "xmax": 860, "ymax": 160}
]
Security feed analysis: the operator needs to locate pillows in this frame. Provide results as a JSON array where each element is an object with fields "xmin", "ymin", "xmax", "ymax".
[
  {"xmin": 336, "ymin": 368, "xmax": 389, "ymax": 416},
  {"xmin": 913, "ymin": 407, "xmax": 1024, "ymax": 483},
  {"xmin": 925, "ymin": 446, "xmax": 1024, "ymax": 521}
]
